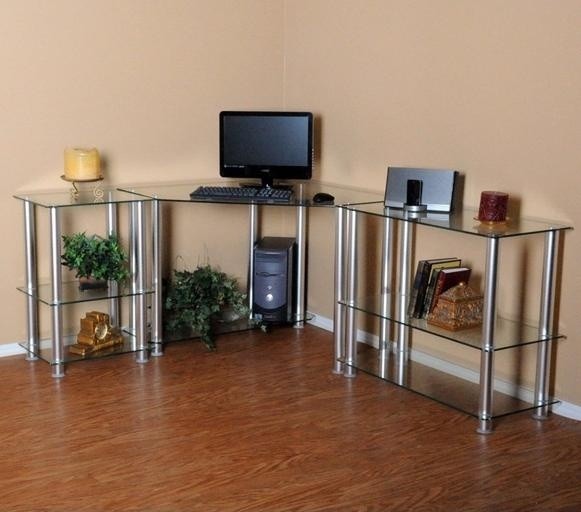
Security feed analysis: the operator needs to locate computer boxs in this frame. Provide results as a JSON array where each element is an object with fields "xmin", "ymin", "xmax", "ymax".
[{"xmin": 253, "ymin": 236, "xmax": 296, "ymax": 327}]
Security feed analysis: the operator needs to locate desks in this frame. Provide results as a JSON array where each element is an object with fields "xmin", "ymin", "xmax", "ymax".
[
  {"xmin": 338, "ymin": 201, "xmax": 573, "ymax": 432},
  {"xmin": 116, "ymin": 180, "xmax": 390, "ymax": 375},
  {"xmin": 12, "ymin": 189, "xmax": 152, "ymax": 378}
]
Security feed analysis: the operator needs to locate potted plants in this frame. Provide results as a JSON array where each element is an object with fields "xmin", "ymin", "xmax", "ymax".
[
  {"xmin": 59, "ymin": 233, "xmax": 134, "ymax": 291},
  {"xmin": 164, "ymin": 266, "xmax": 269, "ymax": 347}
]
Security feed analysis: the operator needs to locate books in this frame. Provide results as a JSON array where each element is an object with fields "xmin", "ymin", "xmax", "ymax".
[{"xmin": 407, "ymin": 256, "xmax": 472, "ymax": 320}]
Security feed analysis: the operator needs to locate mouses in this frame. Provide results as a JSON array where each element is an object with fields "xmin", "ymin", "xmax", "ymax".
[{"xmin": 312, "ymin": 193, "xmax": 335, "ymax": 203}]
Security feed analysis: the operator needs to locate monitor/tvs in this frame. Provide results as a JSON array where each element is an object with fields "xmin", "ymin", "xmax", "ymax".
[{"xmin": 219, "ymin": 110, "xmax": 314, "ymax": 192}]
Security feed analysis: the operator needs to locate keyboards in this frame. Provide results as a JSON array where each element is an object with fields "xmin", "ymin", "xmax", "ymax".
[{"xmin": 188, "ymin": 184, "xmax": 296, "ymax": 202}]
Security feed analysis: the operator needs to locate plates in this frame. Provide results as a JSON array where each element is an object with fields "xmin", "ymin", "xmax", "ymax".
[{"xmin": 473, "ymin": 216, "xmax": 510, "ymax": 225}]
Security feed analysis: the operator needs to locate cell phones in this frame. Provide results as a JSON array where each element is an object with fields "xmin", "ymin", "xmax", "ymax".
[{"xmin": 405, "ymin": 179, "xmax": 422, "ymax": 207}]
